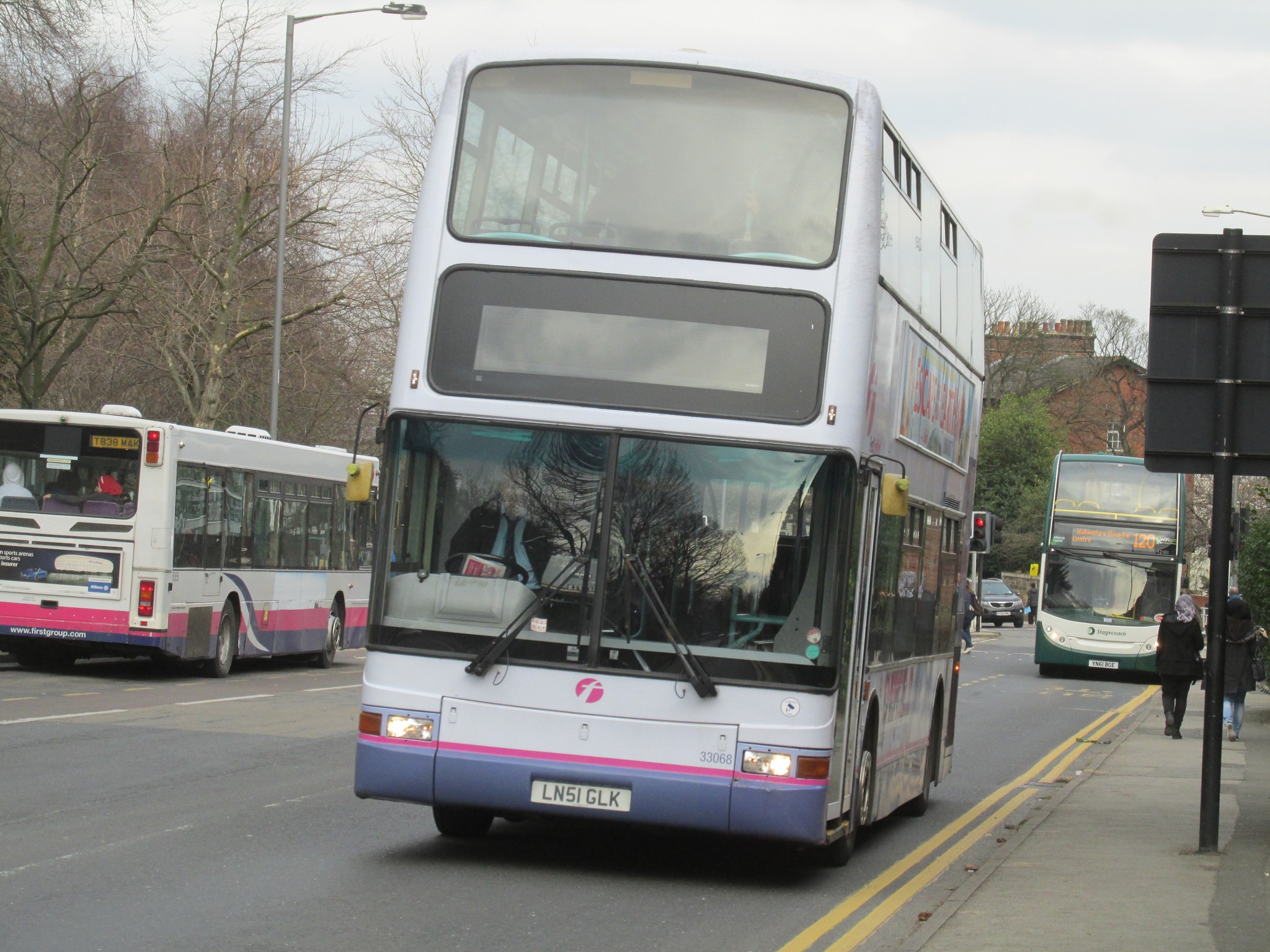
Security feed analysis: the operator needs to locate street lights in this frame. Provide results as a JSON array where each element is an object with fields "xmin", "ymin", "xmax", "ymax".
[{"xmin": 267, "ymin": 2, "xmax": 429, "ymax": 441}]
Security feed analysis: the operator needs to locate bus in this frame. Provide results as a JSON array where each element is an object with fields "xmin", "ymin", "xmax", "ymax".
[
  {"xmin": 339, "ymin": 39, "xmax": 985, "ymax": 867},
  {"xmin": 1032, "ymin": 449, "xmax": 1190, "ymax": 679},
  {"xmin": 1, "ymin": 402, "xmax": 383, "ymax": 681}
]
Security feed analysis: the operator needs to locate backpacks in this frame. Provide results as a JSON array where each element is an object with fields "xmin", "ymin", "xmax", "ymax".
[{"xmin": 967, "ymin": 591, "xmax": 983, "ymax": 616}]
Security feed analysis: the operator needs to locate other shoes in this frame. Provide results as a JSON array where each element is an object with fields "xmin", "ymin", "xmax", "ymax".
[
  {"xmin": 961, "ymin": 650, "xmax": 971, "ymax": 654},
  {"xmin": 963, "ymin": 646, "xmax": 975, "ymax": 654},
  {"xmin": 1227, "ymin": 723, "xmax": 1236, "ymax": 741},
  {"xmin": 1235, "ymin": 735, "xmax": 1239, "ymax": 741},
  {"xmin": 1173, "ymin": 729, "xmax": 1182, "ymax": 739},
  {"xmin": 1164, "ymin": 712, "xmax": 1175, "ymax": 737}
]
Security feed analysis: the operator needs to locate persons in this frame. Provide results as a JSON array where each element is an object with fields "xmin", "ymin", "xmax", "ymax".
[
  {"xmin": 962, "ymin": 579, "xmax": 983, "ymax": 655},
  {"xmin": 444, "ymin": 465, "xmax": 556, "ymax": 601},
  {"xmin": 1203, "ymin": 587, "xmax": 1268, "ymax": 742},
  {"xmin": 1024, "ymin": 583, "xmax": 1039, "ymax": 625},
  {"xmin": 0, "ymin": 462, "xmax": 32, "ymax": 508},
  {"xmin": 43, "ymin": 475, "xmax": 133, "ymax": 517},
  {"xmin": 1156, "ymin": 594, "xmax": 1204, "ymax": 740}
]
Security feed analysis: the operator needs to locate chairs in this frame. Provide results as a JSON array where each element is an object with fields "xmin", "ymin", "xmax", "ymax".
[
  {"xmin": 0, "ymin": 496, "xmax": 136, "ymax": 518},
  {"xmin": 1053, "ymin": 498, "xmax": 1178, "ymax": 524},
  {"xmin": 175, "ymin": 515, "xmax": 240, "ymax": 569},
  {"xmin": 471, "ymin": 218, "xmax": 622, "ymax": 249}
]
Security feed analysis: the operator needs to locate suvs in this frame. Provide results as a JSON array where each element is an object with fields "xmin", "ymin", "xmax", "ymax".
[{"xmin": 966, "ymin": 577, "xmax": 1026, "ymax": 628}]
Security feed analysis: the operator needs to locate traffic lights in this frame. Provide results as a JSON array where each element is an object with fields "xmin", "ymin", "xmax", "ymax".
[
  {"xmin": 990, "ymin": 515, "xmax": 1004, "ymax": 545},
  {"xmin": 970, "ymin": 511, "xmax": 990, "ymax": 552}
]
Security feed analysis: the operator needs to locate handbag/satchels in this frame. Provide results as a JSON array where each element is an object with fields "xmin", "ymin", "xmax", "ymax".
[{"xmin": 1252, "ymin": 647, "xmax": 1266, "ymax": 680}]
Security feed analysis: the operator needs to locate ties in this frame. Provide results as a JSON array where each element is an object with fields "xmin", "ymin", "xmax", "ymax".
[{"xmin": 503, "ymin": 513, "xmax": 524, "ymax": 581}]
